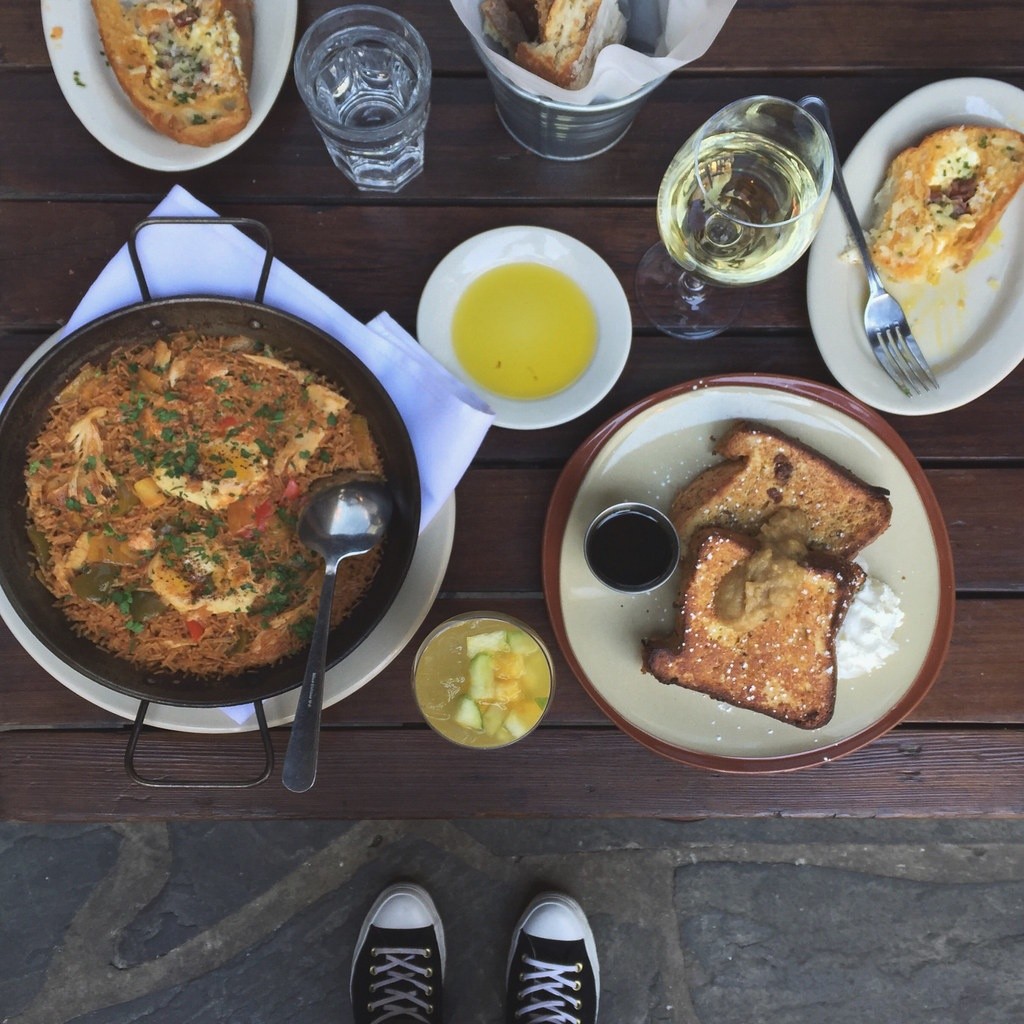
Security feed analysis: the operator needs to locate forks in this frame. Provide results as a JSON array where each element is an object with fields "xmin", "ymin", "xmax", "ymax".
[{"xmin": 795, "ymin": 96, "xmax": 940, "ymax": 398}]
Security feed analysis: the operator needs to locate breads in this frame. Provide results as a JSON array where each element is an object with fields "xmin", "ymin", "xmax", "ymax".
[
  {"xmin": 478, "ymin": 0, "xmax": 629, "ymax": 91},
  {"xmin": 645, "ymin": 529, "xmax": 869, "ymax": 732},
  {"xmin": 91, "ymin": 0, "xmax": 252, "ymax": 148},
  {"xmin": 665, "ymin": 421, "xmax": 892, "ymax": 569},
  {"xmin": 864, "ymin": 125, "xmax": 1024, "ymax": 284}
]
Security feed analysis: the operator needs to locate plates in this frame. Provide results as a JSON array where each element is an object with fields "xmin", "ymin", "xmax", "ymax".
[
  {"xmin": 415, "ymin": 226, "xmax": 632, "ymax": 430},
  {"xmin": 806, "ymin": 77, "xmax": 1023, "ymax": 417},
  {"xmin": 0, "ymin": 321, "xmax": 457, "ymax": 734},
  {"xmin": 41, "ymin": 0, "xmax": 297, "ymax": 174}
]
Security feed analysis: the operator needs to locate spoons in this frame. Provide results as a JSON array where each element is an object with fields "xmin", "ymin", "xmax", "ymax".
[{"xmin": 280, "ymin": 472, "xmax": 398, "ymax": 794}]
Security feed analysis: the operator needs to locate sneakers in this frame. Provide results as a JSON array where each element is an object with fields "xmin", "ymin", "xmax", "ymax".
[
  {"xmin": 506, "ymin": 890, "xmax": 601, "ymax": 1024},
  {"xmin": 349, "ymin": 881, "xmax": 447, "ymax": 1024}
]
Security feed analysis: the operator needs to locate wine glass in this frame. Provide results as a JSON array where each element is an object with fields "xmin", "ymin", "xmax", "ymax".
[{"xmin": 633, "ymin": 94, "xmax": 834, "ymax": 339}]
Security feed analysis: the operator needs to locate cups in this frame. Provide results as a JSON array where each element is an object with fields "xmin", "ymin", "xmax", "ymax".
[
  {"xmin": 469, "ymin": 27, "xmax": 673, "ymax": 161},
  {"xmin": 293, "ymin": 4, "xmax": 432, "ymax": 193},
  {"xmin": 411, "ymin": 610, "xmax": 556, "ymax": 752}
]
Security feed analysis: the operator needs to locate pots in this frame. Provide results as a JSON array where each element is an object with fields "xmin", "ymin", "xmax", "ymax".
[{"xmin": 0, "ymin": 219, "xmax": 421, "ymax": 789}]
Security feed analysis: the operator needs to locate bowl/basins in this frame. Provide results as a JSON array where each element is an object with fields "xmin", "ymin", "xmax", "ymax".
[
  {"xmin": 584, "ymin": 502, "xmax": 682, "ymax": 597},
  {"xmin": 538, "ymin": 372, "xmax": 956, "ymax": 779}
]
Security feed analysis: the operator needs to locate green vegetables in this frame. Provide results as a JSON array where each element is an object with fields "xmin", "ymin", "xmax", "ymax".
[{"xmin": 26, "ymin": 344, "xmax": 339, "ymax": 648}]
